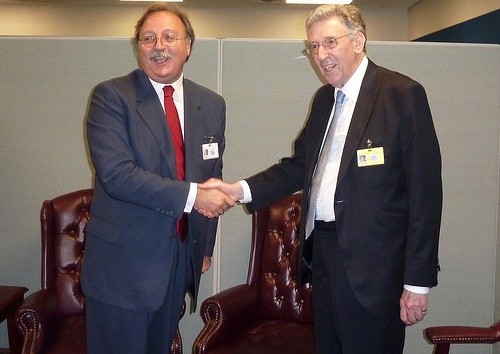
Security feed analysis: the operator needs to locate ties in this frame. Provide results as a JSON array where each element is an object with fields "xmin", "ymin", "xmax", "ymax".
[
  {"xmin": 305, "ymin": 90, "xmax": 345, "ymax": 243},
  {"xmin": 163, "ymin": 85, "xmax": 188, "ymax": 243}
]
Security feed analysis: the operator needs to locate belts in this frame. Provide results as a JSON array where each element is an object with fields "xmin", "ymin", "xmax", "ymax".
[{"xmin": 314, "ymin": 219, "xmax": 336, "ymax": 232}]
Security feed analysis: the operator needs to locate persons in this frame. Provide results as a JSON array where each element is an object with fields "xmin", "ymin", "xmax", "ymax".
[
  {"xmin": 81, "ymin": 3, "xmax": 236, "ymax": 354},
  {"xmin": 194, "ymin": 5, "xmax": 443, "ymax": 354}
]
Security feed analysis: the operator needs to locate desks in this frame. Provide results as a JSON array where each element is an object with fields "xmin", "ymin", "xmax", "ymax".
[{"xmin": 0, "ymin": 286, "xmax": 29, "ymax": 354}]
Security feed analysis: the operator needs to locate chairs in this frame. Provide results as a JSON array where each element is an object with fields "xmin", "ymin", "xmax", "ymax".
[
  {"xmin": 191, "ymin": 193, "xmax": 314, "ymax": 354},
  {"xmin": 14, "ymin": 188, "xmax": 188, "ymax": 354}
]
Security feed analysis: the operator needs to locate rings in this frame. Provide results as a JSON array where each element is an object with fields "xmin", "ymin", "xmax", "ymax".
[
  {"xmin": 421, "ymin": 309, "xmax": 426, "ymax": 312},
  {"xmin": 218, "ymin": 209, "xmax": 224, "ymax": 215}
]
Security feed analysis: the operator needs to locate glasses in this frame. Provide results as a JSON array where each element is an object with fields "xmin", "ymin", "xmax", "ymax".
[
  {"xmin": 306, "ymin": 32, "xmax": 351, "ymax": 54},
  {"xmin": 137, "ymin": 35, "xmax": 187, "ymax": 47}
]
